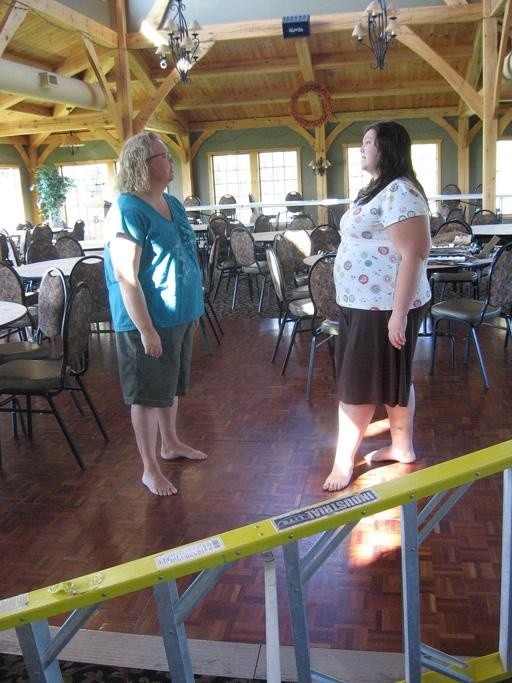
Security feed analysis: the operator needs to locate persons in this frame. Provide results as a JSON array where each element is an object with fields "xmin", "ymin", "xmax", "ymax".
[
  {"xmin": 104, "ymin": 131, "xmax": 208, "ymax": 496},
  {"xmin": 322, "ymin": 120, "xmax": 432, "ymax": 491}
]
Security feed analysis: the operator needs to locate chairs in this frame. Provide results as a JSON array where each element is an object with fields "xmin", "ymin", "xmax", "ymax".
[
  {"xmin": 184, "ymin": 193, "xmax": 349, "ymax": 401},
  {"xmin": 1, "ymin": 217, "xmax": 105, "ymax": 471},
  {"xmin": 418, "ymin": 184, "xmax": 512, "ymax": 390}
]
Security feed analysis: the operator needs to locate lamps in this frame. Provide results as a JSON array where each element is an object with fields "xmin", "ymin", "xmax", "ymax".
[
  {"xmin": 153, "ymin": 0, "xmax": 206, "ymax": 83},
  {"xmin": 307, "ymin": 157, "xmax": 332, "ymax": 176},
  {"xmin": 351, "ymin": 0, "xmax": 403, "ymax": 70},
  {"xmin": 57, "ymin": 104, "xmax": 87, "ymax": 156}
]
